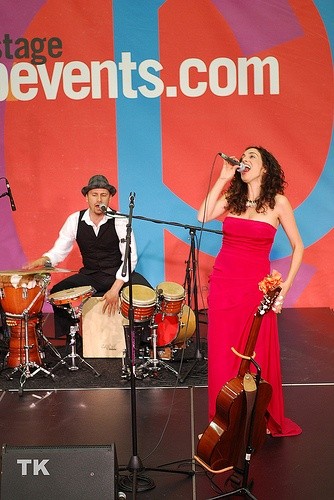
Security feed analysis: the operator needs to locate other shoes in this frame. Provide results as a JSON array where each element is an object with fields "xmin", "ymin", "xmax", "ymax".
[{"xmin": 65, "ymin": 333, "xmax": 81, "ymax": 354}]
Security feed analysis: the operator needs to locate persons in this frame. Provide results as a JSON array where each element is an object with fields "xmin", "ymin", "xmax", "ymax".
[
  {"xmin": 197, "ymin": 146, "xmax": 304, "ymax": 439},
  {"xmin": 22, "ymin": 174, "xmax": 154, "ymax": 356}
]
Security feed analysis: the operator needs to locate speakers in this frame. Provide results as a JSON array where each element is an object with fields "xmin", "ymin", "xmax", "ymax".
[{"xmin": 0, "ymin": 442, "xmax": 116, "ymax": 500}]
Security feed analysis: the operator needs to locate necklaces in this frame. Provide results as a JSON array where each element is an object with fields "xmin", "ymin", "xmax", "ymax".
[{"xmin": 246, "ymin": 198, "xmax": 257, "ymax": 207}]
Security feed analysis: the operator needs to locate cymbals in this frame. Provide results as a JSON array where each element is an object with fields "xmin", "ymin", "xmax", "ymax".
[{"xmin": 31, "ymin": 266, "xmax": 71, "ymax": 274}]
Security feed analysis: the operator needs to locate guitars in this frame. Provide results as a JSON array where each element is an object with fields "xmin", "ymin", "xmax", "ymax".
[{"xmin": 196, "ymin": 284, "xmax": 283, "ymax": 474}]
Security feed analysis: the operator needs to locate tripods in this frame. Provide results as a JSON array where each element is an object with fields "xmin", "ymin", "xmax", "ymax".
[
  {"xmin": 4, "ymin": 271, "xmax": 102, "ymax": 397},
  {"xmin": 116, "ymin": 192, "xmax": 195, "ymax": 500},
  {"xmin": 103, "ymin": 210, "xmax": 224, "ymax": 383},
  {"xmin": 210, "ymin": 347, "xmax": 262, "ymax": 500}
]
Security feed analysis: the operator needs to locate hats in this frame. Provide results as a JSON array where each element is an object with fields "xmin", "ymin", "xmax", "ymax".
[{"xmin": 81, "ymin": 175, "xmax": 117, "ymax": 197}]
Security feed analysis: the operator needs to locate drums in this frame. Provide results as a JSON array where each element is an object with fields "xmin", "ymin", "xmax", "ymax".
[
  {"xmin": 48, "ymin": 285, "xmax": 93, "ymax": 317},
  {"xmin": 0, "ymin": 269, "xmax": 52, "ymax": 371},
  {"xmin": 157, "ymin": 281, "xmax": 185, "ymax": 317},
  {"xmin": 150, "ymin": 302, "xmax": 196, "ymax": 347},
  {"xmin": 119, "ymin": 284, "xmax": 157, "ymax": 323}
]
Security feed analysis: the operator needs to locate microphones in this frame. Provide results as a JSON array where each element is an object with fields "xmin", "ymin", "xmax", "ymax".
[
  {"xmin": 5, "ymin": 178, "xmax": 16, "ymax": 211},
  {"xmin": 218, "ymin": 152, "xmax": 245, "ymax": 172},
  {"xmin": 99, "ymin": 202, "xmax": 106, "ymax": 215}
]
